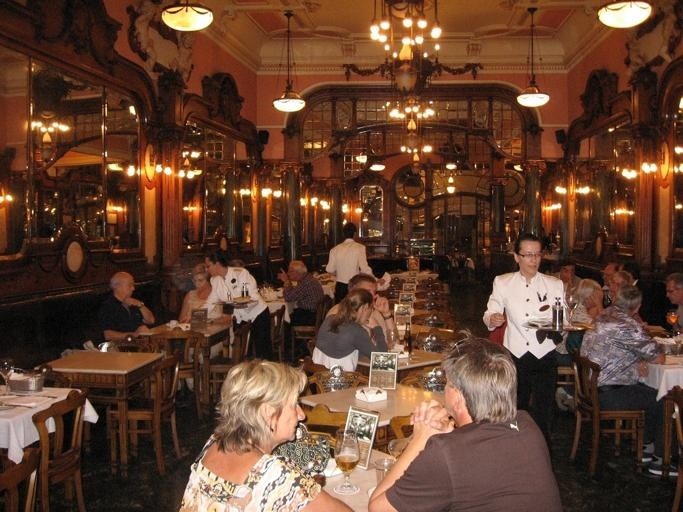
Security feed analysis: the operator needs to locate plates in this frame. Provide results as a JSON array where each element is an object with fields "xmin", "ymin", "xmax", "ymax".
[
  {"xmin": 528, "ymin": 317, "xmax": 552, "ymax": 326},
  {"xmin": 1, "ymin": 396, "xmax": 48, "ymax": 406},
  {"xmin": 233, "ymin": 296, "xmax": 252, "ymax": 304}
]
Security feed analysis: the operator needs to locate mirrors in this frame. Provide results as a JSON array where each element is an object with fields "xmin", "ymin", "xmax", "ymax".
[
  {"xmin": 566, "ymin": 109, "xmax": 639, "ymax": 257},
  {"xmin": 669, "ymin": 82, "xmax": 683, "ymax": 267},
  {"xmin": 0, "ymin": 37, "xmax": 146, "ymax": 267},
  {"xmin": 177, "ymin": 112, "xmax": 260, "ymax": 254},
  {"xmin": 358, "ymin": 185, "xmax": 384, "ymax": 238}
]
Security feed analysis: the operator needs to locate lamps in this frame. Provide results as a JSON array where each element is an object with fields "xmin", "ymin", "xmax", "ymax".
[
  {"xmin": 387, "ymin": 99, "xmax": 436, "ymax": 160},
  {"xmin": 355, "ymin": 100, "xmax": 386, "ymax": 172},
  {"xmin": 444, "ymin": 130, "xmax": 457, "ymax": 195},
  {"xmin": 368, "ymin": 0, "xmax": 443, "ymax": 62},
  {"xmin": 272, "ymin": 14, "xmax": 306, "ymax": 114},
  {"xmin": 516, "ymin": 8, "xmax": 551, "ymax": 108},
  {"xmin": 597, "ymin": 0, "xmax": 652, "ymax": 29},
  {"xmin": 20, "ymin": 110, "xmax": 72, "ymax": 142},
  {"xmin": 160, "ymin": 0, "xmax": 214, "ymax": 33}
]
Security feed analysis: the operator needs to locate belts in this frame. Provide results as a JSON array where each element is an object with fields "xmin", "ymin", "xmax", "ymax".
[{"xmin": 597, "ymin": 385, "xmax": 623, "ymax": 392}]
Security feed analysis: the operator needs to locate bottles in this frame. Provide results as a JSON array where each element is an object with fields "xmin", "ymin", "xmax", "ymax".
[
  {"xmin": 602, "ymin": 286, "xmax": 611, "ymax": 309},
  {"xmin": 227, "ymin": 291, "xmax": 232, "ymax": 301},
  {"xmin": 552, "ymin": 297, "xmax": 564, "ymax": 328},
  {"xmin": 402, "ymin": 322, "xmax": 413, "ymax": 355},
  {"xmin": 241, "ymin": 282, "xmax": 248, "ymax": 298}
]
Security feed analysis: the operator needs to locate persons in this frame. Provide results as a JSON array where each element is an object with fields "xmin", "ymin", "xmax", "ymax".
[
  {"xmin": 324, "ymin": 273, "xmax": 401, "ymax": 345},
  {"xmin": 483, "ymin": 234, "xmax": 569, "ymax": 437},
  {"xmin": 367, "ymin": 327, "xmax": 566, "ymax": 511},
  {"xmin": 312, "ymin": 287, "xmax": 388, "ymax": 373},
  {"xmin": 277, "ymin": 259, "xmax": 324, "ymax": 364},
  {"xmin": 179, "ymin": 262, "xmax": 235, "ymax": 392},
  {"xmin": 103, "ymin": 271, "xmax": 155, "ymax": 341},
  {"xmin": 548, "ymin": 258, "xmax": 683, "ymax": 477},
  {"xmin": 178, "ymin": 356, "xmax": 355, "ymax": 512},
  {"xmin": 325, "ymin": 222, "xmax": 386, "ymax": 303},
  {"xmin": 179, "ymin": 246, "xmax": 274, "ymax": 361}
]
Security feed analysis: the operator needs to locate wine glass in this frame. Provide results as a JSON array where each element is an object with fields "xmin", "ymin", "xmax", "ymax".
[
  {"xmin": 0, "ymin": 357, "xmax": 16, "ymax": 398},
  {"xmin": 563, "ymin": 293, "xmax": 580, "ymax": 328},
  {"xmin": 673, "ymin": 329, "xmax": 683, "ymax": 358},
  {"xmin": 669, "ymin": 311, "xmax": 679, "ymax": 337},
  {"xmin": 334, "ymin": 429, "xmax": 361, "ymax": 496}
]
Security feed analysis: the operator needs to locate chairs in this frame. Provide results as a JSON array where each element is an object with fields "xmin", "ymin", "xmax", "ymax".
[
  {"xmin": 529, "ymin": 366, "xmax": 582, "ymax": 416},
  {"xmin": 668, "ymin": 383, "xmax": 683, "ymax": 512},
  {"xmin": 569, "ymin": 347, "xmax": 645, "ymax": 477},
  {"xmin": 1, "ymin": 269, "xmax": 458, "ymax": 511}
]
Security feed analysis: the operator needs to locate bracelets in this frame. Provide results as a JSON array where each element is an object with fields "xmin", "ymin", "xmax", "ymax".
[
  {"xmin": 137, "ymin": 300, "xmax": 145, "ymax": 308},
  {"xmin": 384, "ymin": 313, "xmax": 393, "ymax": 321}
]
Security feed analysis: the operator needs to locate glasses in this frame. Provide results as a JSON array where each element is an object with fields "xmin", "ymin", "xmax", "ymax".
[
  {"xmin": 451, "ymin": 338, "xmax": 489, "ymax": 356},
  {"xmin": 516, "ymin": 252, "xmax": 543, "ymax": 258}
]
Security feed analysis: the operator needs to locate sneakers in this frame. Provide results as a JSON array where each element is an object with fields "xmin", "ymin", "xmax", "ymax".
[
  {"xmin": 649, "ymin": 462, "xmax": 679, "ymax": 477},
  {"xmin": 642, "ymin": 452, "xmax": 655, "ymax": 463}
]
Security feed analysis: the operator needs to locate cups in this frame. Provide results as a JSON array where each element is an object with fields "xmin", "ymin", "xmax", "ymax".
[{"xmin": 375, "ymin": 459, "xmax": 395, "ymax": 487}]
